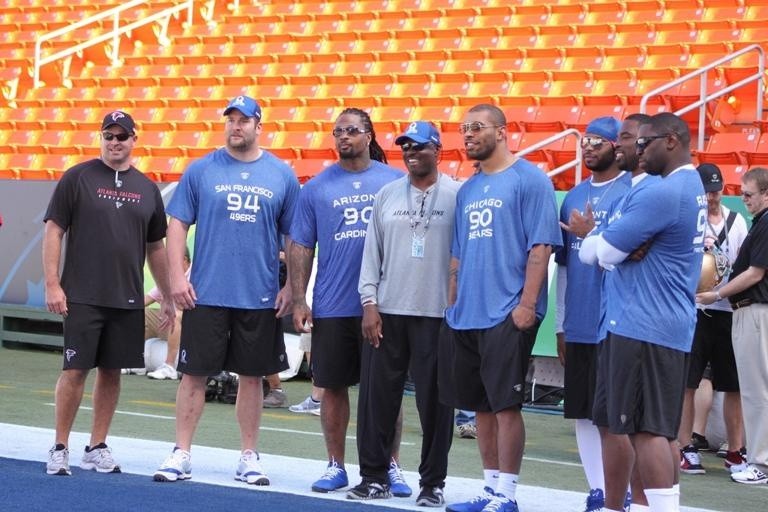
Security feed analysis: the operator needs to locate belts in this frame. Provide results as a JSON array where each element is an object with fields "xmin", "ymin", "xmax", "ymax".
[{"xmin": 731, "ymin": 299, "xmax": 752, "ymax": 309}]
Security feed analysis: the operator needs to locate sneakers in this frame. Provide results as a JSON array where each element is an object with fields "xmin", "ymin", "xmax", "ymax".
[
  {"xmin": 45, "ymin": 445, "xmax": 72, "ymax": 475},
  {"xmin": 347, "ymin": 459, "xmax": 445, "ymax": 507},
  {"xmin": 122, "ymin": 363, "xmax": 179, "ymax": 379},
  {"xmin": 458, "ymin": 421, "xmax": 477, "ymax": 440},
  {"xmin": 311, "ymin": 461, "xmax": 349, "ymax": 493},
  {"xmin": 680, "ymin": 437, "xmax": 710, "ymax": 473},
  {"xmin": 580, "ymin": 488, "xmax": 633, "ymax": 512},
  {"xmin": 153, "ymin": 449, "xmax": 192, "ymax": 482},
  {"xmin": 288, "ymin": 398, "xmax": 320, "ymax": 416},
  {"xmin": 446, "ymin": 487, "xmax": 518, "ymax": 511},
  {"xmin": 264, "ymin": 390, "xmax": 287, "ymax": 408},
  {"xmin": 233, "ymin": 449, "xmax": 269, "ymax": 486},
  {"xmin": 718, "ymin": 441, "xmax": 768, "ymax": 484},
  {"xmin": 79, "ymin": 443, "xmax": 119, "ymax": 474}
]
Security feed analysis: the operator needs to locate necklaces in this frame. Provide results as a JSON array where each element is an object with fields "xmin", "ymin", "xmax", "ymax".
[{"xmin": 406, "ymin": 178, "xmax": 438, "ymax": 239}]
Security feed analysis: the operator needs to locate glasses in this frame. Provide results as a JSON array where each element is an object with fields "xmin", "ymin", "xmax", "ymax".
[
  {"xmin": 739, "ymin": 191, "xmax": 761, "ymax": 196},
  {"xmin": 580, "ymin": 135, "xmax": 610, "ymax": 148},
  {"xmin": 635, "ymin": 135, "xmax": 669, "ymax": 150},
  {"xmin": 457, "ymin": 123, "xmax": 499, "ymax": 133},
  {"xmin": 102, "ymin": 131, "xmax": 132, "ymax": 142},
  {"xmin": 333, "ymin": 127, "xmax": 370, "ymax": 135},
  {"xmin": 401, "ymin": 141, "xmax": 433, "ymax": 151}
]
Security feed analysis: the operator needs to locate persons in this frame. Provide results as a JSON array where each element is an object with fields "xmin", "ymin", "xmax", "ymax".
[
  {"xmin": 345, "ymin": 120, "xmax": 463, "ymax": 507},
  {"xmin": 558, "ymin": 112, "xmax": 652, "ymax": 512},
  {"xmin": 289, "ymin": 107, "xmax": 413, "ymax": 496},
  {"xmin": 678, "ymin": 164, "xmax": 768, "ymax": 484},
  {"xmin": 143, "ymin": 242, "xmax": 192, "ymax": 379},
  {"xmin": 153, "ymin": 96, "xmax": 300, "ymax": 486},
  {"xmin": 290, "ymin": 334, "xmax": 322, "ymax": 417},
  {"xmin": 453, "ymin": 407, "xmax": 477, "ymax": 440},
  {"xmin": 579, "ymin": 112, "xmax": 706, "ymax": 512},
  {"xmin": 43, "ymin": 112, "xmax": 175, "ymax": 476},
  {"xmin": 438, "ymin": 103, "xmax": 563, "ymax": 512},
  {"xmin": 262, "ymin": 254, "xmax": 287, "ymax": 409}
]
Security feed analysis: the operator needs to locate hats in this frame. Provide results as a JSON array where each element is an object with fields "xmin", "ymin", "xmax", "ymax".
[
  {"xmin": 587, "ymin": 117, "xmax": 623, "ymax": 148},
  {"xmin": 697, "ymin": 163, "xmax": 723, "ymax": 193},
  {"xmin": 395, "ymin": 121, "xmax": 441, "ymax": 146},
  {"xmin": 103, "ymin": 111, "xmax": 136, "ymax": 132},
  {"xmin": 223, "ymin": 96, "xmax": 262, "ymax": 120}
]
